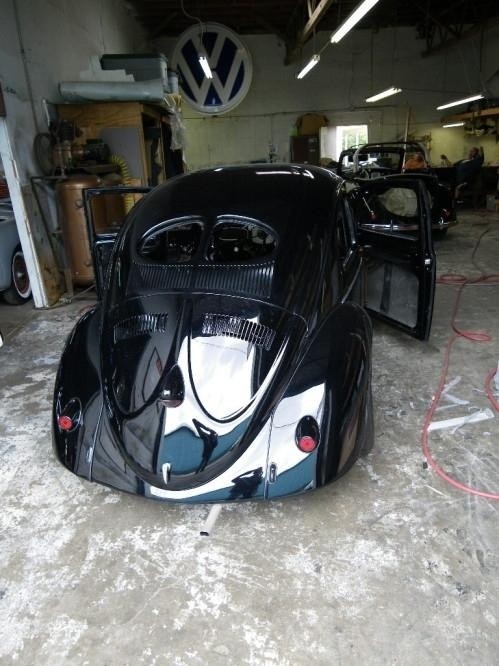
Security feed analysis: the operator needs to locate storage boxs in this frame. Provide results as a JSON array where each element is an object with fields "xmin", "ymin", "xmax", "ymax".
[{"xmin": 101, "ymin": 52, "xmax": 170, "ymax": 88}]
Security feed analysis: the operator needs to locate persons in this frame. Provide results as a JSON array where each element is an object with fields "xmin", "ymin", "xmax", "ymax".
[
  {"xmin": 441, "ymin": 153, "xmax": 453, "ymax": 166},
  {"xmin": 468, "ymin": 145, "xmax": 483, "ymax": 159},
  {"xmin": 405, "ymin": 151, "xmax": 430, "ymax": 168}
]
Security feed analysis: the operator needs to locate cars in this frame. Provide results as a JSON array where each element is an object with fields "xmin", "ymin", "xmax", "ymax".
[
  {"xmin": 52, "ymin": 162, "xmax": 435, "ymax": 504},
  {"xmin": 337, "ymin": 141, "xmax": 456, "ymax": 245}
]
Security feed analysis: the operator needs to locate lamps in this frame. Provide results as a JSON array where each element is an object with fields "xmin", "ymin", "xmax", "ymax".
[
  {"xmin": 296, "ymin": 51, "xmax": 320, "ymax": 79},
  {"xmin": 180, "ymin": 0, "xmax": 213, "ymax": 79},
  {"xmin": 435, "ymin": 27, "xmax": 485, "ymax": 111},
  {"xmin": 441, "ymin": 119, "xmax": 466, "ymax": 130},
  {"xmin": 329, "ymin": 0, "xmax": 376, "ymax": 45},
  {"xmin": 365, "ymin": 28, "xmax": 402, "ymax": 103}
]
